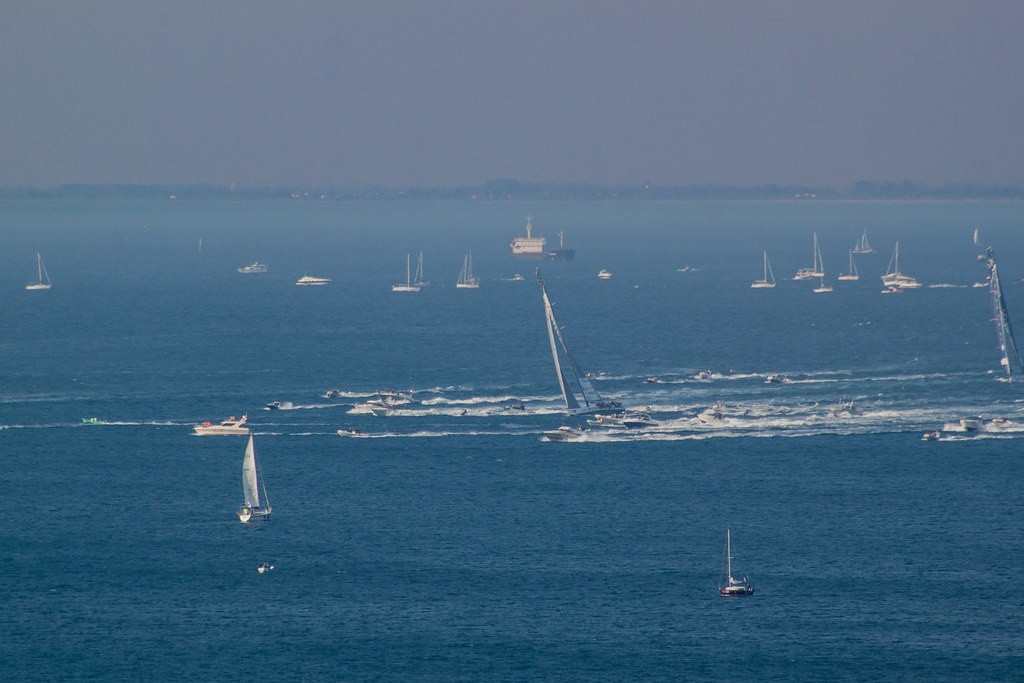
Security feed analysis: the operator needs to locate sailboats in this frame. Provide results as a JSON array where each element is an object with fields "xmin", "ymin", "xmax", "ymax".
[
  {"xmin": 509, "ymin": 215, "xmax": 576, "ymax": 261},
  {"xmin": 236, "ymin": 433, "xmax": 273, "ymax": 522},
  {"xmin": 538, "ymin": 280, "xmax": 624, "ymax": 415},
  {"xmin": 27, "ymin": 252, "xmax": 53, "ymax": 290},
  {"xmin": 392, "ymin": 253, "xmax": 433, "ymax": 292},
  {"xmin": 721, "ymin": 532, "xmax": 756, "ymax": 597},
  {"xmin": 455, "ymin": 250, "xmax": 480, "ymax": 289},
  {"xmin": 751, "ymin": 229, "xmax": 917, "ymax": 292}
]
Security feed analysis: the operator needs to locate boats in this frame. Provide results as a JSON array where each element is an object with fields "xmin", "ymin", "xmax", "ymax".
[
  {"xmin": 768, "ymin": 374, "xmax": 786, "ymax": 384},
  {"xmin": 921, "ymin": 415, "xmax": 1015, "ymax": 442},
  {"xmin": 648, "ymin": 376, "xmax": 659, "ymax": 384},
  {"xmin": 192, "ymin": 414, "xmax": 252, "ymax": 435},
  {"xmin": 266, "ymin": 401, "xmax": 284, "ymax": 410},
  {"xmin": 80, "ymin": 417, "xmax": 105, "ymax": 425},
  {"xmin": 328, "ymin": 385, "xmax": 525, "ymax": 437},
  {"xmin": 239, "ymin": 264, "xmax": 268, "ymax": 273},
  {"xmin": 296, "ymin": 278, "xmax": 330, "ymax": 286},
  {"xmin": 693, "ymin": 370, "xmax": 714, "ymax": 381},
  {"xmin": 594, "ymin": 408, "xmax": 660, "ymax": 428},
  {"xmin": 544, "ymin": 423, "xmax": 588, "ymax": 441}
]
